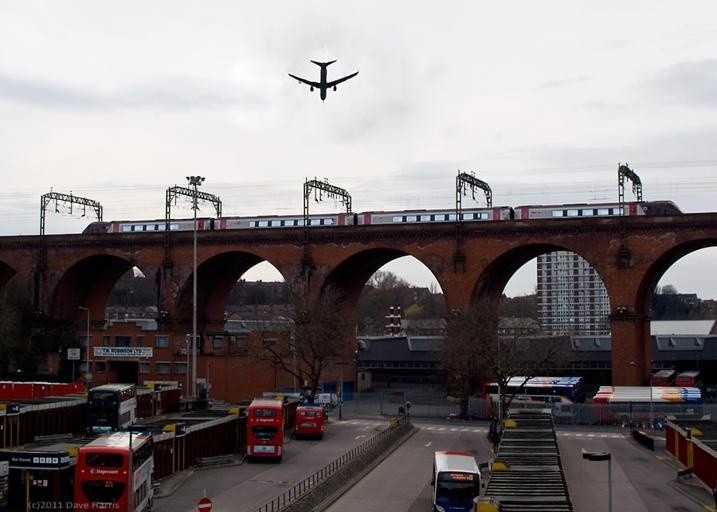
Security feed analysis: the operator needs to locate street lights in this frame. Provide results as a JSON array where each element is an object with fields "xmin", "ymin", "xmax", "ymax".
[
  {"xmin": 78, "ymin": 306, "xmax": 90, "ymax": 373},
  {"xmin": 582, "ymin": 452, "xmax": 613, "ymax": 512},
  {"xmin": 186, "ymin": 176, "xmax": 205, "ymax": 394}
]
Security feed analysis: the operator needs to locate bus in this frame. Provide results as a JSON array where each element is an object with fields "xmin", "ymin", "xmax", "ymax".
[
  {"xmin": 86, "ymin": 382, "xmax": 137, "ymax": 432},
  {"xmin": 245, "ymin": 398, "xmax": 327, "ymax": 463},
  {"xmin": 73, "ymin": 432, "xmax": 155, "ymax": 512},
  {"xmin": 481, "ymin": 370, "xmax": 704, "ymax": 427}
]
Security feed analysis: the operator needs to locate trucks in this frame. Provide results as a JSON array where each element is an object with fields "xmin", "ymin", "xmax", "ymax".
[{"xmin": 431, "ymin": 450, "xmax": 485, "ymax": 512}]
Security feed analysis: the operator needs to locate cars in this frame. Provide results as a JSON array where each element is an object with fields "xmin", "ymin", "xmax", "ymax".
[{"xmin": 302, "ymin": 393, "xmax": 338, "ymax": 409}]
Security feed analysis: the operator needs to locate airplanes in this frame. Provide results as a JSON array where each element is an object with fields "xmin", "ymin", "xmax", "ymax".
[{"xmin": 289, "ymin": 61, "xmax": 358, "ymax": 100}]
